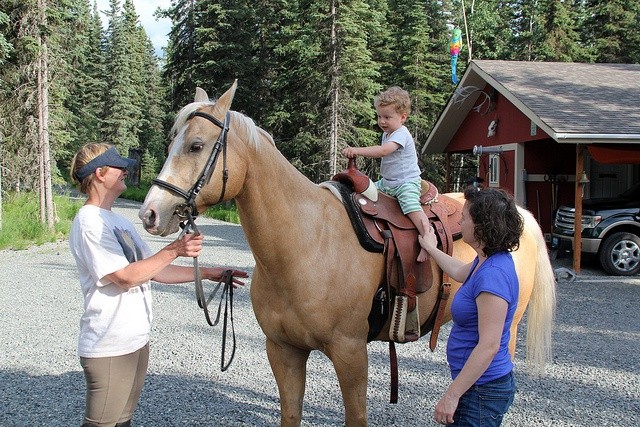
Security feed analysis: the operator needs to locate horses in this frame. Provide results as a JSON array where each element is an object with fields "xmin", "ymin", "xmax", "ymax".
[{"xmin": 139, "ymin": 79, "xmax": 558, "ymax": 427}]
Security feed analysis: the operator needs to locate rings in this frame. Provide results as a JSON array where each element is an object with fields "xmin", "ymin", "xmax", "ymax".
[{"xmin": 441, "ymin": 420, "xmax": 444, "ymax": 423}]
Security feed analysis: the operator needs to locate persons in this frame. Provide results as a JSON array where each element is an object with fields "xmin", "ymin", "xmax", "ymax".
[
  {"xmin": 342, "ymin": 85, "xmax": 438, "ymax": 263},
  {"xmin": 68, "ymin": 142, "xmax": 249, "ymax": 427},
  {"xmin": 417, "ymin": 183, "xmax": 525, "ymax": 427}
]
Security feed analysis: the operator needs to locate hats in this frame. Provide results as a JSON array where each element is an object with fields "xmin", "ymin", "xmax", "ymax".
[{"xmin": 75, "ymin": 147, "xmax": 138, "ymax": 181}]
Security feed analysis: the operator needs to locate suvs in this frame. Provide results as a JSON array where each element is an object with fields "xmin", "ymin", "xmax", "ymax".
[{"xmin": 550, "ymin": 184, "xmax": 640, "ymax": 275}]
